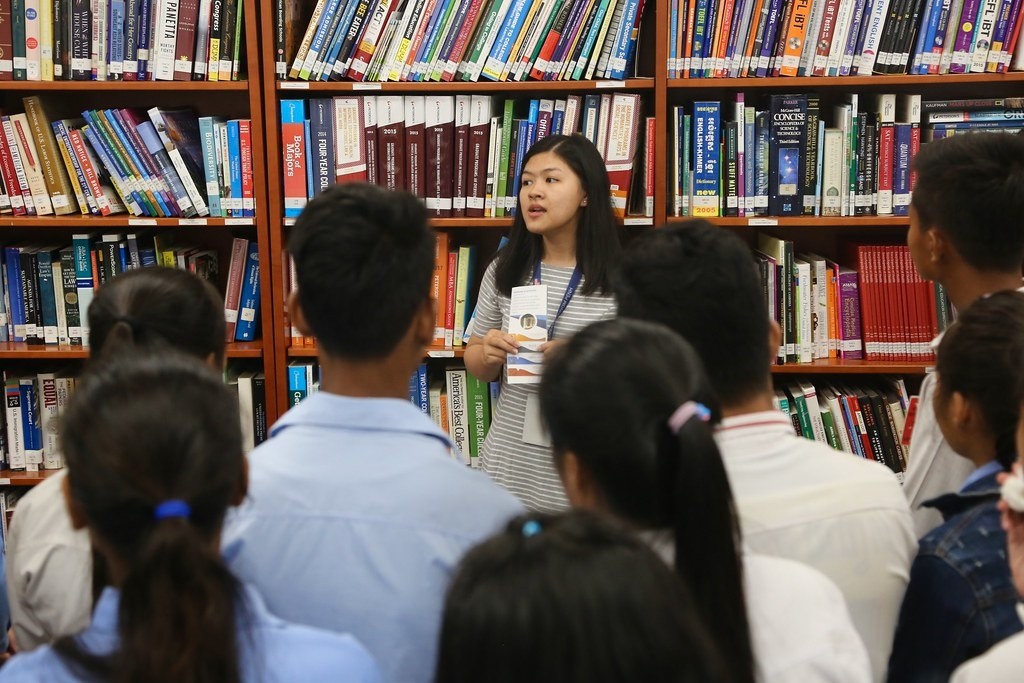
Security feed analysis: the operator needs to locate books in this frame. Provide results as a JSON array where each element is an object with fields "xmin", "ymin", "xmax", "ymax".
[{"xmin": 0, "ymin": 1, "xmax": 1024, "ymax": 544}]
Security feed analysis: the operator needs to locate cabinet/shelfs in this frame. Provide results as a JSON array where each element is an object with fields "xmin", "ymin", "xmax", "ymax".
[{"xmin": 0, "ymin": 0, "xmax": 1024, "ymax": 485}]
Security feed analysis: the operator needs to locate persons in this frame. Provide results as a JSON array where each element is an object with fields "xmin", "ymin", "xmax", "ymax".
[
  {"xmin": 2, "ymin": 134, "xmax": 1024, "ymax": 683},
  {"xmin": 0, "ymin": 351, "xmax": 380, "ymax": 683},
  {"xmin": 433, "ymin": 510, "xmax": 737, "ymax": 683}
]
